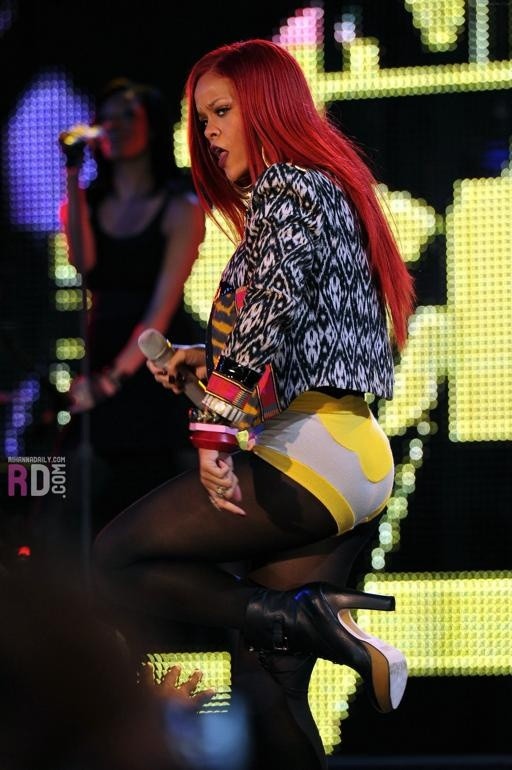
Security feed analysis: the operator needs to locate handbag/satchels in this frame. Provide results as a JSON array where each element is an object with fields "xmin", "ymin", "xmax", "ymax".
[{"xmin": 102, "ymin": 359, "xmax": 129, "ymax": 387}]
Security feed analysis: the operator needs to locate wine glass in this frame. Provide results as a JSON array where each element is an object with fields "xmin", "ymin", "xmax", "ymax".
[{"xmin": 218, "ymin": 484, "xmax": 226, "ymax": 495}]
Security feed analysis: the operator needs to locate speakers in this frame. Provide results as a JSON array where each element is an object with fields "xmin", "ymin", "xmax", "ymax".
[{"xmin": 242, "ymin": 581, "xmax": 408, "ymax": 714}]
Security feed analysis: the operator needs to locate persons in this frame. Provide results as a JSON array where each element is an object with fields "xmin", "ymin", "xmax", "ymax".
[
  {"xmin": 115, "ymin": 661, "xmax": 216, "ymax": 768},
  {"xmin": 92, "ymin": 39, "xmax": 417, "ymax": 769},
  {"xmin": 57, "ymin": 73, "xmax": 204, "ymax": 438}
]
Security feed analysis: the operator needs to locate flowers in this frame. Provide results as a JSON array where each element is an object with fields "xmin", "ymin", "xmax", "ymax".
[{"xmin": 137, "ymin": 329, "xmax": 206, "ymax": 410}]
